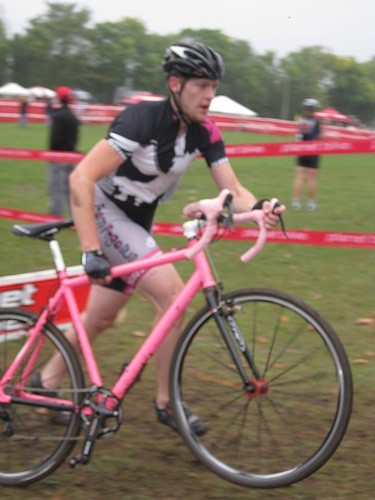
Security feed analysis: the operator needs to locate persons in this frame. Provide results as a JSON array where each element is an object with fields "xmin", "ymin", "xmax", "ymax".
[
  {"xmin": 47, "ymin": 96, "xmax": 81, "ymax": 218},
  {"xmin": 25, "ymin": 40, "xmax": 287, "ymax": 437},
  {"xmin": 19, "ymin": 102, "xmax": 28, "ymax": 127},
  {"xmin": 292, "ymin": 98, "xmax": 330, "ymax": 212}
]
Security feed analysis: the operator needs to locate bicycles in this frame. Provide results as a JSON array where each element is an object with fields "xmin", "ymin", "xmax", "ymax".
[{"xmin": 0, "ymin": 188, "xmax": 354, "ymax": 490}]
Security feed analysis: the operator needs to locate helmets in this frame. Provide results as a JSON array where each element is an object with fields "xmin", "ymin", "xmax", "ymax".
[
  {"xmin": 162, "ymin": 38, "xmax": 226, "ymax": 80},
  {"xmin": 301, "ymin": 98, "xmax": 323, "ymax": 109}
]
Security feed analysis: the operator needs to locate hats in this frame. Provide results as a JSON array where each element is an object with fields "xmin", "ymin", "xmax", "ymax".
[{"xmin": 56, "ymin": 85, "xmax": 76, "ymax": 104}]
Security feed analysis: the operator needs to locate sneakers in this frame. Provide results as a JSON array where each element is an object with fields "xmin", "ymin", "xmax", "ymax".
[
  {"xmin": 152, "ymin": 396, "xmax": 208, "ymax": 438},
  {"xmin": 23, "ymin": 370, "xmax": 74, "ymax": 426}
]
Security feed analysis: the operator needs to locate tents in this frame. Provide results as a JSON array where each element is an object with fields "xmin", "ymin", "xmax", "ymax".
[
  {"xmin": 208, "ymin": 95, "xmax": 258, "ymax": 117},
  {"xmin": 119, "ymin": 93, "xmax": 168, "ymax": 105},
  {"xmin": 312, "ymin": 106, "xmax": 352, "ymax": 127},
  {"xmin": 0, "ymin": 82, "xmax": 95, "ymax": 103}
]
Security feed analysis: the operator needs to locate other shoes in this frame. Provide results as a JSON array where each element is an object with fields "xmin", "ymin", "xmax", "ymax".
[
  {"xmin": 306, "ymin": 200, "xmax": 318, "ymax": 212},
  {"xmin": 291, "ymin": 199, "xmax": 302, "ymax": 211}
]
